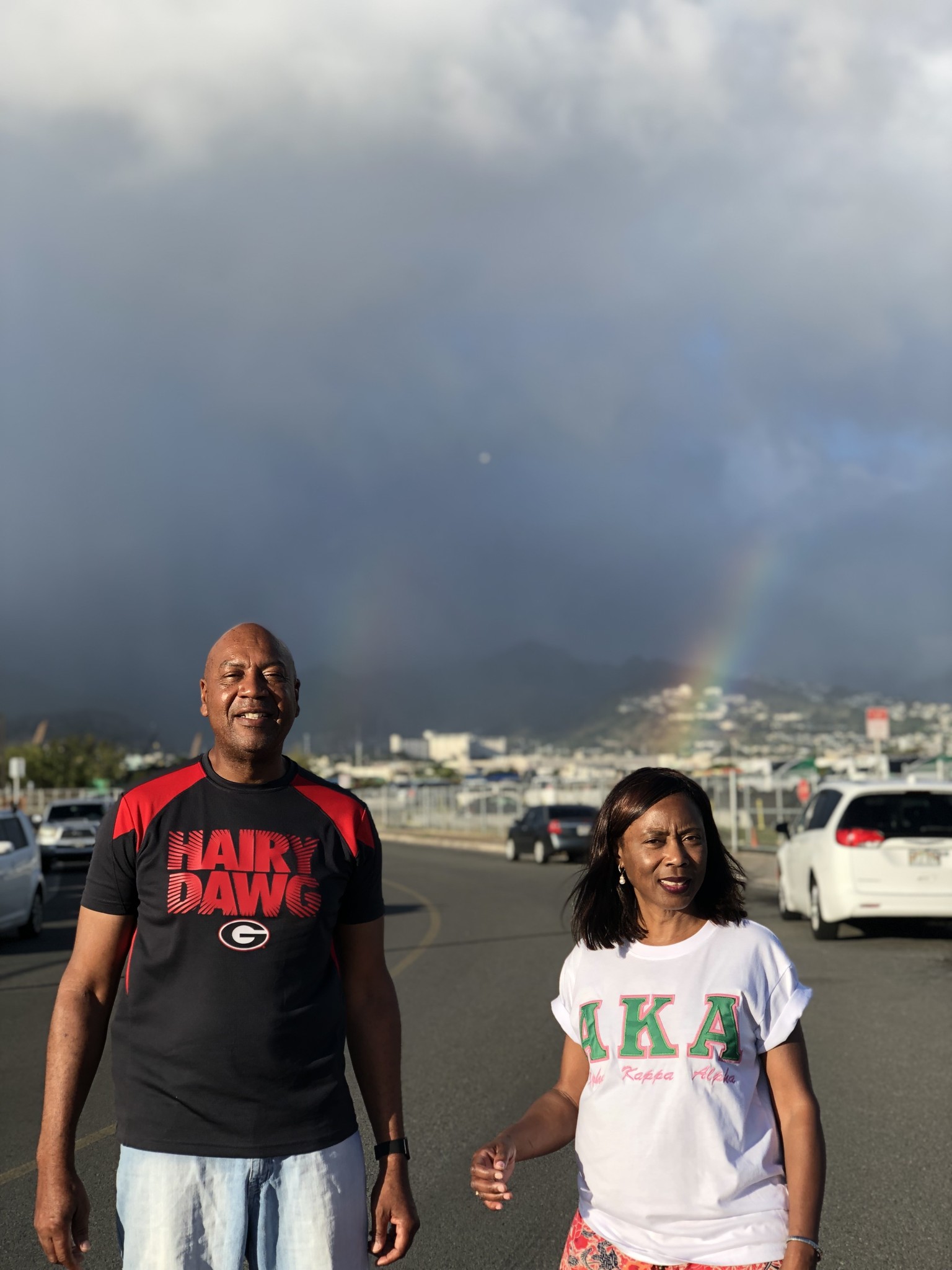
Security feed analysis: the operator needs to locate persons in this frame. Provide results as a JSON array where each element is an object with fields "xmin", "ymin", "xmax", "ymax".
[
  {"xmin": 469, "ymin": 765, "xmax": 827, "ymax": 1270},
  {"xmin": 31, "ymin": 622, "xmax": 419, "ymax": 1270}
]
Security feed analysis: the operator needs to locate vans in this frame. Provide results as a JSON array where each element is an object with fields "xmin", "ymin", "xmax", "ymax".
[{"xmin": 0, "ymin": 807, "xmax": 46, "ymax": 941}]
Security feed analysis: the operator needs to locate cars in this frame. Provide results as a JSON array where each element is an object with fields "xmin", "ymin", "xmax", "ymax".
[
  {"xmin": 773, "ymin": 776, "xmax": 952, "ymax": 940},
  {"xmin": 506, "ymin": 803, "xmax": 602, "ymax": 864}
]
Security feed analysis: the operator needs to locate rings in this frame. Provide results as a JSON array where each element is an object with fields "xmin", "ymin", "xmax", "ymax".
[{"xmin": 474, "ymin": 1190, "xmax": 482, "ymax": 1201}]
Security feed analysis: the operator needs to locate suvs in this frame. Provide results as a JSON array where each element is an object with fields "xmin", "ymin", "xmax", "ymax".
[{"xmin": 32, "ymin": 796, "xmax": 117, "ymax": 866}]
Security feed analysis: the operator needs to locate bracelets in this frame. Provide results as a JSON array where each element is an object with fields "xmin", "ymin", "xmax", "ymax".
[
  {"xmin": 786, "ymin": 1235, "xmax": 823, "ymax": 1263},
  {"xmin": 373, "ymin": 1136, "xmax": 411, "ymax": 1161}
]
